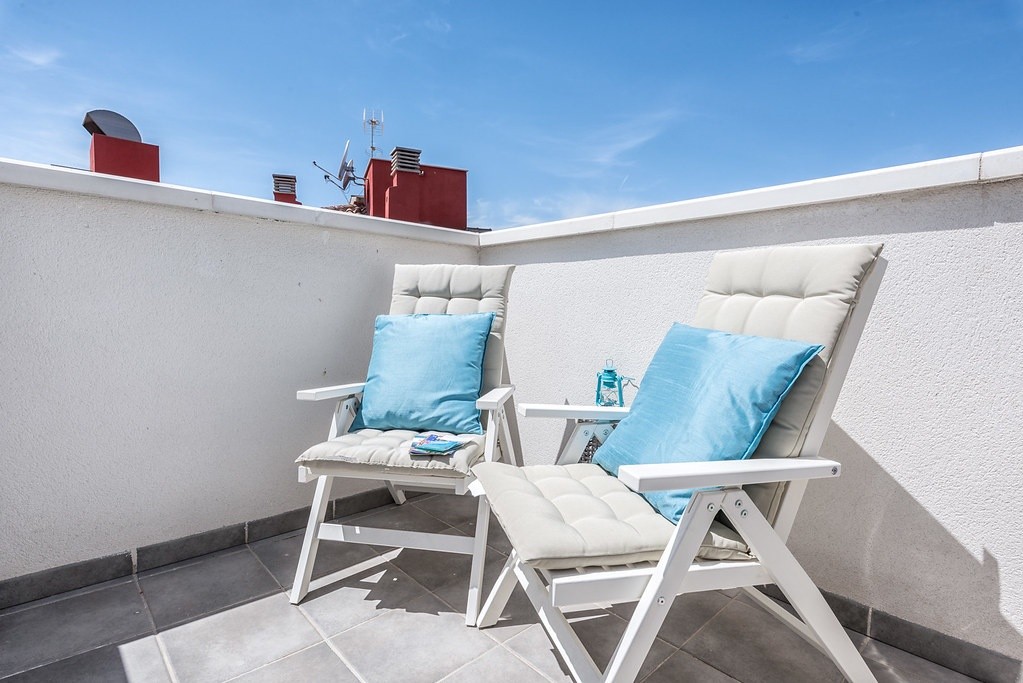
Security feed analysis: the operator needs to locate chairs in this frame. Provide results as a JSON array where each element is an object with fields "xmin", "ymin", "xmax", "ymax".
[
  {"xmin": 288, "ymin": 263, "xmax": 517, "ymax": 627},
  {"xmin": 472, "ymin": 243, "xmax": 882, "ymax": 683}
]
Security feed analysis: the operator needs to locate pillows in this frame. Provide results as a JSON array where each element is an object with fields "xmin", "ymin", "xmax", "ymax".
[
  {"xmin": 348, "ymin": 311, "xmax": 494, "ymax": 434},
  {"xmin": 591, "ymin": 321, "xmax": 824, "ymax": 527}
]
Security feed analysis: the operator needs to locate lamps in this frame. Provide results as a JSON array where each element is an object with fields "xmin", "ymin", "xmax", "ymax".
[{"xmin": 595, "ymin": 358, "xmax": 626, "ymax": 407}]
